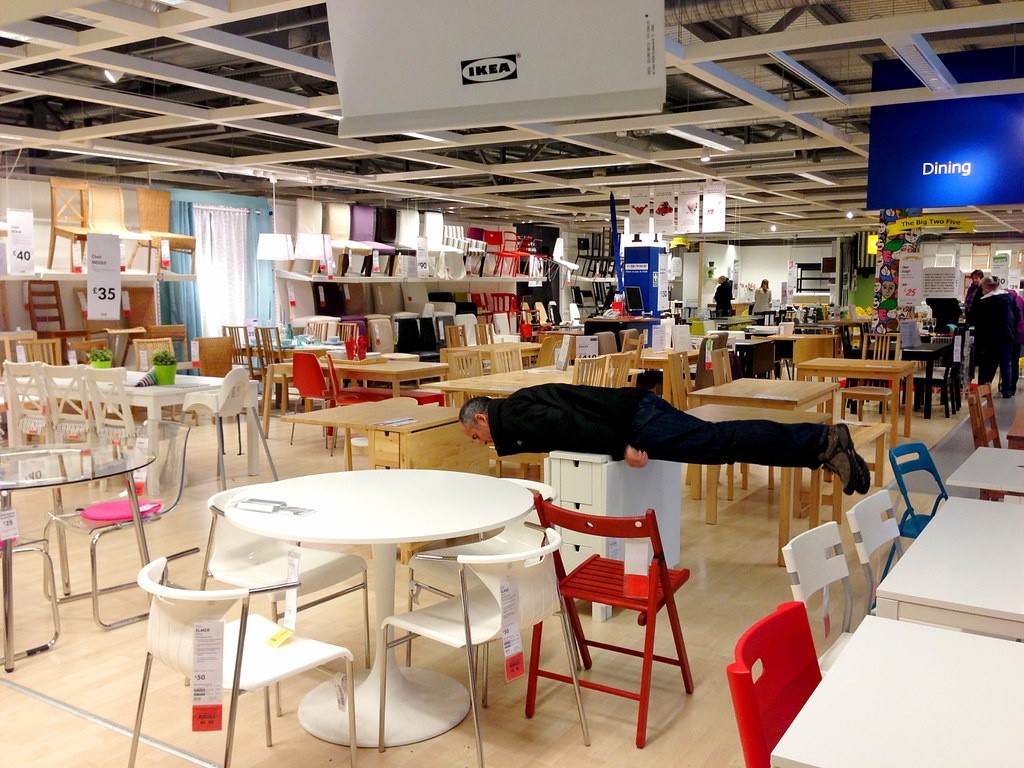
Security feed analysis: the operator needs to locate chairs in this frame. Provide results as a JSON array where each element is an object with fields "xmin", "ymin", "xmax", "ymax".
[{"xmin": 0, "ymin": 177, "xmax": 1024, "ymax": 768}]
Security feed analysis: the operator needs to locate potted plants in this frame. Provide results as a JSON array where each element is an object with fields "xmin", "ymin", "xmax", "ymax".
[
  {"xmin": 152, "ymin": 348, "xmax": 177, "ymax": 385},
  {"xmin": 88, "ymin": 345, "xmax": 113, "ymax": 368}
]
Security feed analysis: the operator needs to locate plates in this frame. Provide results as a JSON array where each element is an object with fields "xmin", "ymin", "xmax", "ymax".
[
  {"xmin": 274, "ymin": 344, "xmax": 296, "ymax": 349},
  {"xmin": 244, "ymin": 344, "xmax": 263, "ymax": 347},
  {"xmin": 324, "ymin": 341, "xmax": 344, "ymax": 345}
]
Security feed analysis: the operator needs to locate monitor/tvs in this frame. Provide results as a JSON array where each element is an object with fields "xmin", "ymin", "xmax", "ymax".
[{"xmin": 624, "ymin": 286, "xmax": 644, "ymax": 311}]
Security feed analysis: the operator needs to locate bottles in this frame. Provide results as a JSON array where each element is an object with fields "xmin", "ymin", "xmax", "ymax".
[{"xmin": 285, "ymin": 324, "xmax": 293, "ymax": 340}]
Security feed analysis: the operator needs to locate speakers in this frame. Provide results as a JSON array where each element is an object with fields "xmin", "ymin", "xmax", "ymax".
[{"xmin": 578, "ymin": 238, "xmax": 589, "ymax": 250}]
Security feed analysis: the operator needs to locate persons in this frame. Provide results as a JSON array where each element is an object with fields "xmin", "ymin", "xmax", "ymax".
[
  {"xmin": 458, "ymin": 383, "xmax": 870, "ymax": 495},
  {"xmin": 962, "ymin": 270, "xmax": 1024, "ymax": 398},
  {"xmin": 752, "ymin": 279, "xmax": 772, "ymax": 315},
  {"xmin": 714, "ymin": 276, "xmax": 732, "ymax": 318}
]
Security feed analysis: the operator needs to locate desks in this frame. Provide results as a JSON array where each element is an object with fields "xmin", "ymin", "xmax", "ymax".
[
  {"xmin": 726, "ymin": 338, "xmax": 772, "ymax": 380},
  {"xmin": 538, "ymin": 331, "xmax": 584, "ymax": 364},
  {"xmin": 688, "ymin": 377, "xmax": 840, "ymax": 502},
  {"xmin": 768, "ymin": 334, "xmax": 836, "ymax": 377},
  {"xmin": 1007, "ymin": 410, "xmax": 1024, "ymax": 450},
  {"xmin": 234, "ymin": 343, "xmax": 330, "ymax": 410},
  {"xmin": 684, "ymin": 404, "xmax": 834, "ymax": 569},
  {"xmin": 574, "ymin": 317, "xmax": 654, "ymax": 354},
  {"xmin": 1, "ymin": 369, "xmax": 261, "ymax": 482},
  {"xmin": 769, "ymin": 616, "xmax": 1024, "ymax": 768},
  {"xmin": 945, "ymin": 445, "xmax": 1024, "ymax": 504},
  {"xmin": 420, "ymin": 364, "xmax": 643, "ymax": 410},
  {"xmin": 264, "ymin": 356, "xmax": 449, "ymax": 440},
  {"xmin": 875, "ymin": 497, "xmax": 1024, "ymax": 640},
  {"xmin": 794, "ymin": 356, "xmax": 916, "ymax": 448},
  {"xmin": 616, "ymin": 349, "xmax": 700, "ymax": 372},
  {"xmin": 223, "ymin": 470, "xmax": 537, "ymax": 750},
  {"xmin": 862, "ymin": 341, "xmax": 952, "ymax": 418},
  {"xmin": 440, "ymin": 342, "xmax": 541, "ymax": 380},
  {"xmin": 279, "ymin": 400, "xmax": 460, "ymax": 470},
  {"xmin": 0, "ymin": 444, "xmax": 157, "ymax": 673}
]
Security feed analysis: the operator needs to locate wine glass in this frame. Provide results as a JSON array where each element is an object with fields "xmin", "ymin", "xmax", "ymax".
[
  {"xmin": 931, "ymin": 318, "xmax": 937, "ymax": 334},
  {"xmin": 925, "ymin": 318, "xmax": 931, "ymax": 333}
]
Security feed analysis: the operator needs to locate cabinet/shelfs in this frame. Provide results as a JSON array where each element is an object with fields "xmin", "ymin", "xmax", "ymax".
[
  {"xmin": 274, "ymin": 267, "xmax": 548, "ymax": 357},
  {"xmin": 369, "ymin": 424, "xmax": 487, "ymax": 563},
  {"xmin": 571, "ymin": 273, "xmax": 624, "ymax": 311},
  {"xmin": 795, "ymin": 261, "xmax": 836, "ymax": 294},
  {"xmin": 0, "ymin": 260, "xmax": 199, "ymax": 371},
  {"xmin": 542, "ymin": 447, "xmax": 683, "ymax": 622}
]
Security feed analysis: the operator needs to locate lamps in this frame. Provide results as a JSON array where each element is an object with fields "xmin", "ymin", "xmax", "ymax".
[
  {"xmin": 700, "ymin": 146, "xmax": 709, "ymax": 161},
  {"xmin": 294, "ymin": 172, "xmax": 335, "ymax": 260},
  {"xmin": 256, "ymin": 176, "xmax": 295, "ymax": 261},
  {"xmin": 104, "ymin": 69, "xmax": 124, "ymax": 83}
]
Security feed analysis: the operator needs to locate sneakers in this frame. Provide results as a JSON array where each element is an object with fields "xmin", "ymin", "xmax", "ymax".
[{"xmin": 817, "ymin": 424, "xmax": 871, "ymax": 495}]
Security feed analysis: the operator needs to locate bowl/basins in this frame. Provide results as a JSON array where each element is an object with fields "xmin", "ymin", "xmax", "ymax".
[
  {"xmin": 329, "ymin": 336, "xmax": 340, "ymax": 342},
  {"xmin": 250, "ymin": 339, "xmax": 261, "ymax": 344},
  {"xmin": 280, "ymin": 338, "xmax": 291, "ymax": 346}
]
